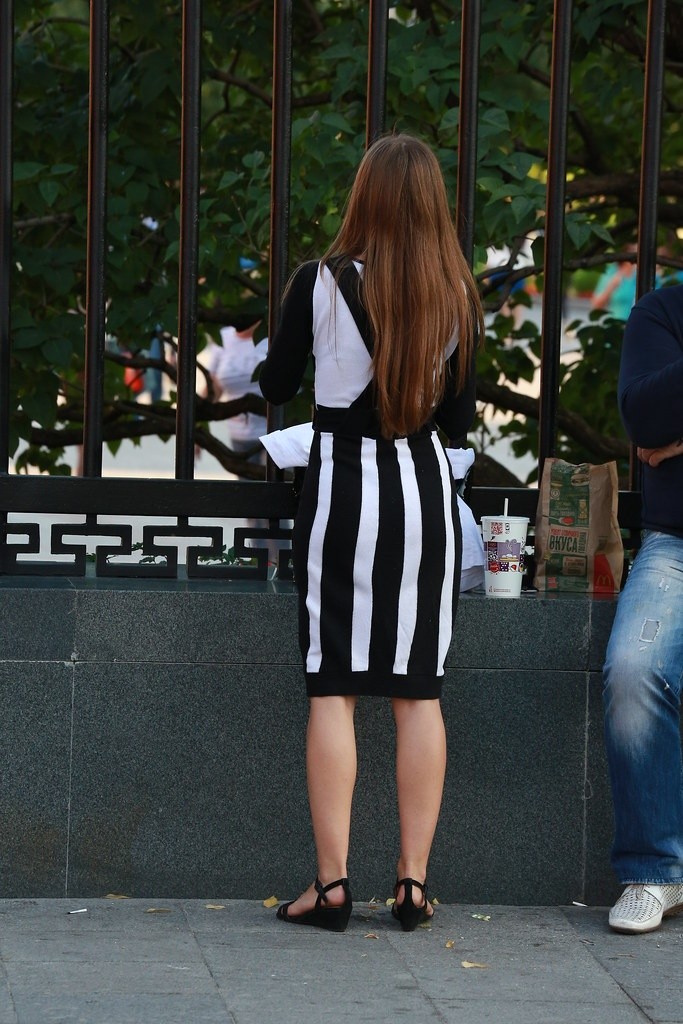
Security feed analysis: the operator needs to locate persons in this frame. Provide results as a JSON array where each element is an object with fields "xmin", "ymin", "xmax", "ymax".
[
  {"xmin": 593, "ymin": 235, "xmax": 683, "ymax": 327},
  {"xmin": 249, "ymin": 136, "xmax": 483, "ymax": 930},
  {"xmin": 487, "ymin": 266, "xmax": 526, "ymax": 347},
  {"xmin": 195, "ymin": 317, "xmax": 291, "ymax": 565},
  {"xmin": 600, "ymin": 279, "xmax": 683, "ymax": 934},
  {"xmin": 108, "ymin": 325, "xmax": 165, "ymax": 422}
]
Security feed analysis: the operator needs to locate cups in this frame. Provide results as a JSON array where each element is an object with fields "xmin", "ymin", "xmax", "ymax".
[{"xmin": 481, "ymin": 515, "xmax": 531, "ymax": 597}]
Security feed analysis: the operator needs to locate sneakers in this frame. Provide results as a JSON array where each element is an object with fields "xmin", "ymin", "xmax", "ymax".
[{"xmin": 608, "ymin": 883, "xmax": 683, "ymax": 932}]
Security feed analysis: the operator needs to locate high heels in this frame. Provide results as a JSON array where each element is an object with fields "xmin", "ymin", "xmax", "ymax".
[
  {"xmin": 276, "ymin": 874, "xmax": 352, "ymax": 932},
  {"xmin": 391, "ymin": 876, "xmax": 434, "ymax": 932}
]
